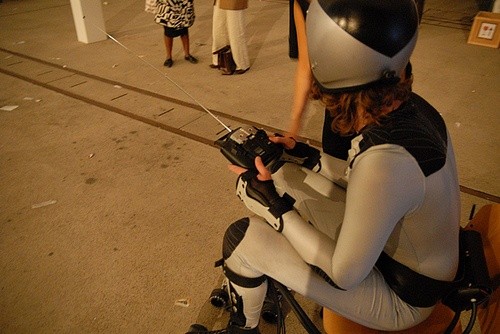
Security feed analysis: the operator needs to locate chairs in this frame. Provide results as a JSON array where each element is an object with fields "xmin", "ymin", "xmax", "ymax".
[{"xmin": 323, "ymin": 202, "xmax": 500, "ymax": 334}]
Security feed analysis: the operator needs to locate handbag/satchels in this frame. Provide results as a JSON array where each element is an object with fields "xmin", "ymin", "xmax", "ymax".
[{"xmin": 213, "ymin": 45, "xmax": 236, "ymax": 75}]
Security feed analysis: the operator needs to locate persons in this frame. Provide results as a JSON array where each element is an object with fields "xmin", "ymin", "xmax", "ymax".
[
  {"xmin": 153, "ymin": 0, "xmax": 198, "ymax": 68},
  {"xmin": 185, "ymin": 0, "xmax": 461, "ymax": 334},
  {"xmin": 208, "ymin": 0, "xmax": 252, "ymax": 75}
]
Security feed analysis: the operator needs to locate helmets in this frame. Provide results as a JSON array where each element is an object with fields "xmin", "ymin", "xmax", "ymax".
[{"xmin": 306, "ymin": 0, "xmax": 419, "ymax": 89}]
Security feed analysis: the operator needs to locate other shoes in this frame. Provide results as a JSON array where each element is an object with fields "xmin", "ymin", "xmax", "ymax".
[
  {"xmin": 236, "ymin": 67, "xmax": 249, "ymax": 74},
  {"xmin": 210, "ymin": 64, "xmax": 219, "ymax": 68}
]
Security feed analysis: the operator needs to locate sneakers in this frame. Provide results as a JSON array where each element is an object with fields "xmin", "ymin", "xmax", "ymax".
[
  {"xmin": 185, "ymin": 55, "xmax": 197, "ymax": 64},
  {"xmin": 208, "ymin": 324, "xmax": 260, "ymax": 334},
  {"xmin": 164, "ymin": 59, "xmax": 172, "ymax": 67}
]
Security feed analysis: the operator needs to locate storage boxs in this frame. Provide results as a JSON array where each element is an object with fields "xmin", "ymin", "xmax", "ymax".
[{"xmin": 466, "ymin": 11, "xmax": 500, "ymax": 48}]
